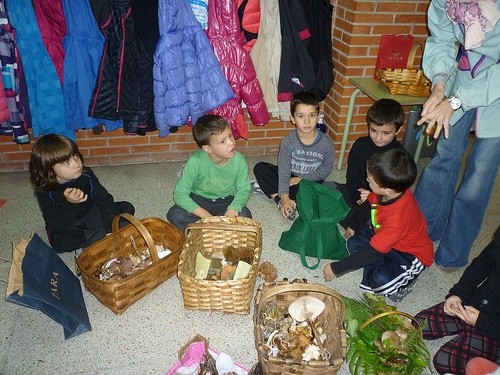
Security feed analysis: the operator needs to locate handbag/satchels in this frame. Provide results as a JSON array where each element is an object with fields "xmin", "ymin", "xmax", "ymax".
[
  {"xmin": 278, "ymin": 178, "xmax": 352, "ymax": 271},
  {"xmin": 373, "ymin": 34, "xmax": 414, "ymax": 81},
  {"xmin": 0, "ymin": 231, "xmax": 91, "ymax": 340}
]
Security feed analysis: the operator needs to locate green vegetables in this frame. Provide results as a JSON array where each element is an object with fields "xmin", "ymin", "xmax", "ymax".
[{"xmin": 339, "ymin": 291, "xmax": 431, "ymax": 375}]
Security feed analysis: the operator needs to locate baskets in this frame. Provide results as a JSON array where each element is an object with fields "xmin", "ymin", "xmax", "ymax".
[
  {"xmin": 177, "ymin": 216, "xmax": 262, "ymax": 315},
  {"xmin": 377, "ymin": 41, "xmax": 432, "ymax": 97},
  {"xmin": 164, "ymin": 334, "xmax": 249, "ymax": 375},
  {"xmin": 77, "ymin": 213, "xmax": 185, "ymax": 315},
  {"xmin": 254, "ymin": 281, "xmax": 346, "ymax": 375},
  {"xmin": 354, "ymin": 305, "xmax": 423, "ymax": 375}
]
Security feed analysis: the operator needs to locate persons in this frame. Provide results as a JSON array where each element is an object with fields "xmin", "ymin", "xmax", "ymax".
[
  {"xmin": 29, "ymin": 134, "xmax": 135, "ymax": 254},
  {"xmin": 324, "ymin": 148, "xmax": 435, "ymax": 302},
  {"xmin": 411, "ymin": 225, "xmax": 500, "ymax": 375},
  {"xmin": 413, "ymin": 0, "xmax": 500, "ymax": 271},
  {"xmin": 167, "ymin": 115, "xmax": 252, "ymax": 233},
  {"xmin": 250, "ymin": 92, "xmax": 336, "ymax": 221},
  {"xmin": 336, "ymin": 99, "xmax": 405, "ymax": 238}
]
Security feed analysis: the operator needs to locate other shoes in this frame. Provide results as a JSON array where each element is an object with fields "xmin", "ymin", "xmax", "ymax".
[
  {"xmin": 388, "ymin": 277, "xmax": 418, "ymax": 302},
  {"xmin": 75, "ymin": 247, "xmax": 86, "ymax": 277}
]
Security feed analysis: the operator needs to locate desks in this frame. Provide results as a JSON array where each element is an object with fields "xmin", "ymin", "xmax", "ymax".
[{"xmin": 337, "ymin": 78, "xmax": 433, "ymax": 169}]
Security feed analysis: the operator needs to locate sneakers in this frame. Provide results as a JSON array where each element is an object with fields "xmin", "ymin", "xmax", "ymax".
[
  {"xmin": 250, "ymin": 180, "xmax": 264, "ymax": 194},
  {"xmin": 277, "ymin": 199, "xmax": 296, "ymax": 221}
]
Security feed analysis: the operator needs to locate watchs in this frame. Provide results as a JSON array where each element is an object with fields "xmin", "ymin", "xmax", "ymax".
[{"xmin": 448, "ymin": 96, "xmax": 461, "ymax": 111}]
